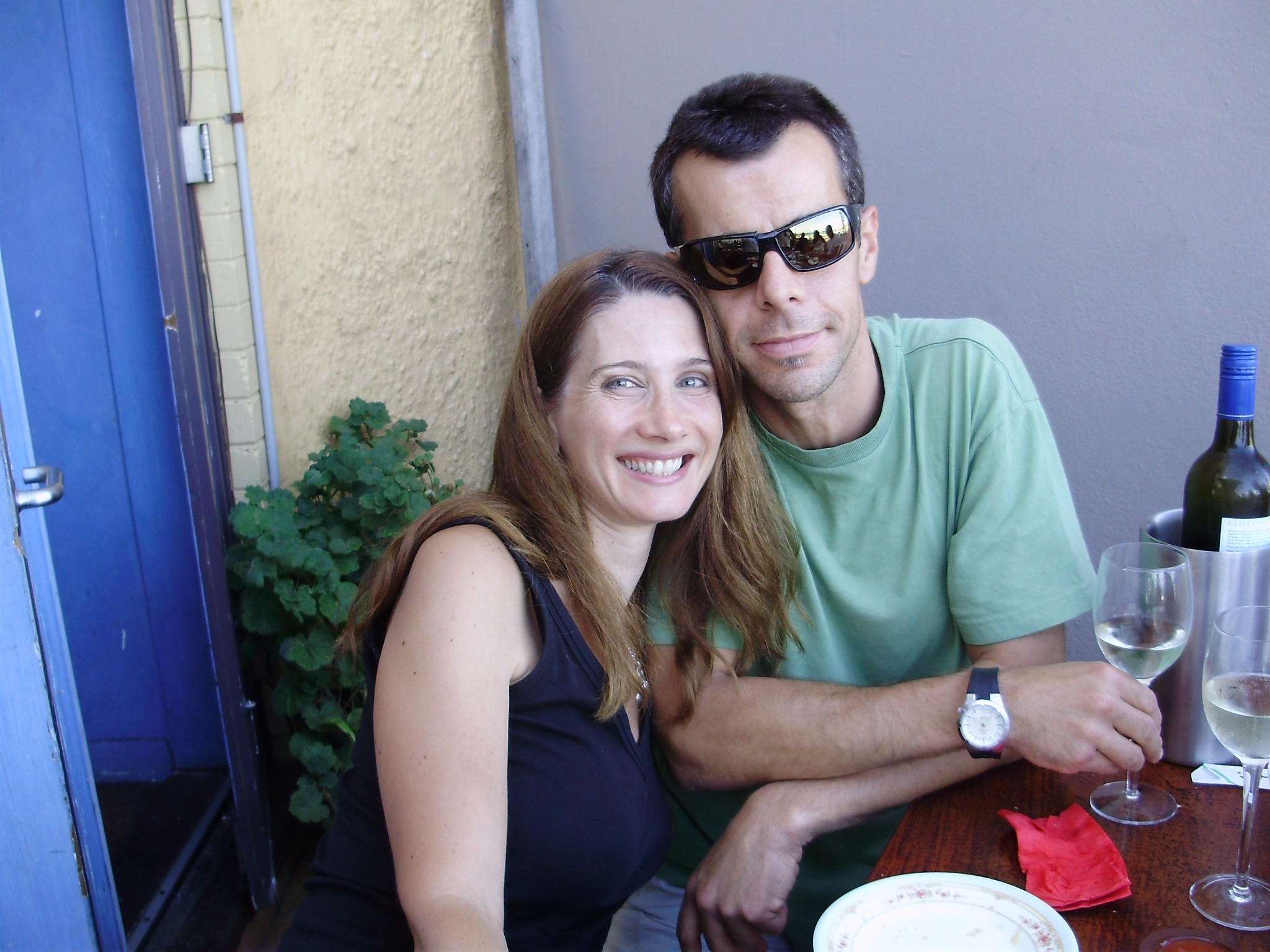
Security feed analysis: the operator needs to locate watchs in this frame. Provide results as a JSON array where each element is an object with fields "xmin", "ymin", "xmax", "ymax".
[{"xmin": 957, "ymin": 667, "xmax": 1009, "ymax": 760}]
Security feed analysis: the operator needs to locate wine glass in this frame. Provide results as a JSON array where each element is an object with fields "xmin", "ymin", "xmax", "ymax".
[
  {"xmin": 1188, "ymin": 606, "xmax": 1270, "ymax": 932},
  {"xmin": 1089, "ymin": 542, "xmax": 1194, "ymax": 825}
]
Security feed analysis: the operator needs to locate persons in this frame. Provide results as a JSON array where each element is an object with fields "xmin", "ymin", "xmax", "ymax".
[
  {"xmin": 280, "ymin": 247, "xmax": 814, "ymax": 952},
  {"xmin": 601, "ymin": 71, "xmax": 1163, "ymax": 952}
]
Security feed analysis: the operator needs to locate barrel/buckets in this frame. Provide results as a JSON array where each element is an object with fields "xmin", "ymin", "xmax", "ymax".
[{"xmin": 1136, "ymin": 507, "xmax": 1269, "ymax": 766}]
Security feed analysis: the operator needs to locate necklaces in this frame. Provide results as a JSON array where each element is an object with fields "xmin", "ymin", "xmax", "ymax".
[{"xmin": 626, "ymin": 645, "xmax": 649, "ymax": 704}]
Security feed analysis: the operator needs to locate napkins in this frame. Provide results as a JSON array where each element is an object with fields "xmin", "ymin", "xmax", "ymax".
[{"xmin": 997, "ymin": 802, "xmax": 1133, "ymax": 911}]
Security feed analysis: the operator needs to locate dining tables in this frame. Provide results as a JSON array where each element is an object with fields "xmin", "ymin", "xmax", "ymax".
[{"xmin": 869, "ymin": 753, "xmax": 1270, "ymax": 952}]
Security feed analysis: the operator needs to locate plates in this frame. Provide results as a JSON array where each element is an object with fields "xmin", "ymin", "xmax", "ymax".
[{"xmin": 813, "ymin": 871, "xmax": 1079, "ymax": 952}]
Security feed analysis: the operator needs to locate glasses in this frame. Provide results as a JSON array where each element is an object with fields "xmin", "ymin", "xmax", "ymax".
[{"xmin": 674, "ymin": 202, "xmax": 864, "ymax": 290}]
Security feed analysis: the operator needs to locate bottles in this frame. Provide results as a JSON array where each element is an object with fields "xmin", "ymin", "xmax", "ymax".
[{"xmin": 1180, "ymin": 343, "xmax": 1269, "ymax": 552}]
